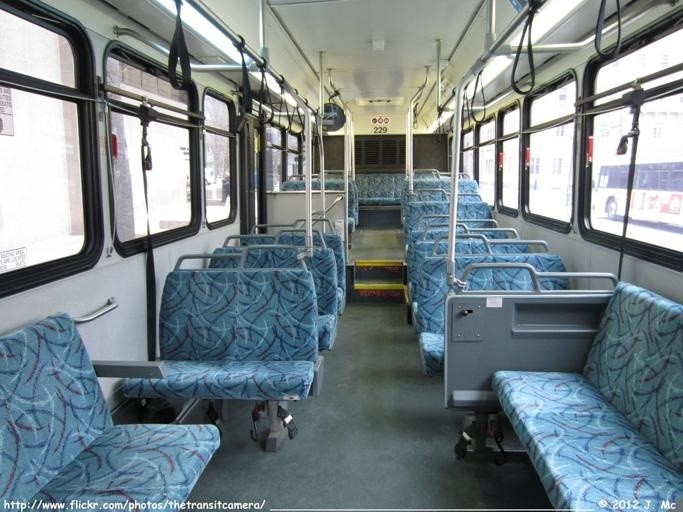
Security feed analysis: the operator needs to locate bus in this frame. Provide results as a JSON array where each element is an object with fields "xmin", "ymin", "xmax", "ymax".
[{"xmin": 591, "ymin": 162, "xmax": 683, "ymax": 230}]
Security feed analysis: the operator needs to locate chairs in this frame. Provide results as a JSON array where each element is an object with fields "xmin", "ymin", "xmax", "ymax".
[{"xmin": 0, "ymin": 173, "xmax": 683, "ymax": 512}]
